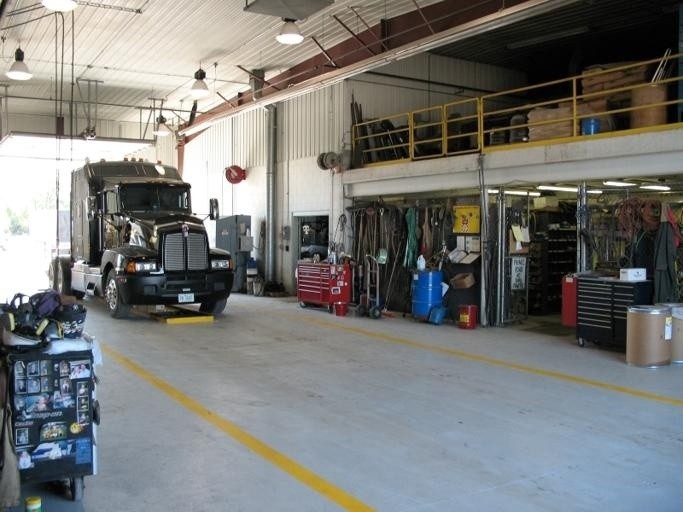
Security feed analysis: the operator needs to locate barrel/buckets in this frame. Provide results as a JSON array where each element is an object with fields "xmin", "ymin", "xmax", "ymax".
[
  {"xmin": 625, "ymin": 305, "xmax": 674, "ymax": 368},
  {"xmin": 657, "ymin": 301, "xmax": 682, "ymax": 363},
  {"xmin": 458, "ymin": 304, "xmax": 479, "ymax": 328},
  {"xmin": 560, "ymin": 276, "xmax": 577, "ymax": 327},
  {"xmin": 412, "ymin": 268, "xmax": 443, "ymax": 318}
]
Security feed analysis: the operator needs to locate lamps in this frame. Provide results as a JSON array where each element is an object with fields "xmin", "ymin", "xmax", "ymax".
[
  {"xmin": 275, "ymin": 17, "xmax": 305, "ymax": 44},
  {"xmin": 188, "ymin": 59, "xmax": 211, "ymax": 98},
  {"xmin": 5, "ymin": 40, "xmax": 34, "ymax": 81}
]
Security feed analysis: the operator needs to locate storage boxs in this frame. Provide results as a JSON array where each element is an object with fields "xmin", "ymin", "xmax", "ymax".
[{"xmin": 533, "ymin": 196, "xmax": 559, "ymax": 212}]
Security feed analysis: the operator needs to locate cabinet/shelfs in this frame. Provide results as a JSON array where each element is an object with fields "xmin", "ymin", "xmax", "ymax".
[
  {"xmin": 297, "ymin": 261, "xmax": 352, "ymax": 308},
  {"xmin": 514, "ymin": 211, "xmax": 576, "ymax": 316},
  {"xmin": 575, "ymin": 273, "xmax": 654, "ymax": 348}
]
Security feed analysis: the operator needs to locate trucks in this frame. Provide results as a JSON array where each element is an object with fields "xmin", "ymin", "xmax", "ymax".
[{"xmin": 45, "ymin": 156, "xmax": 235, "ymax": 318}]
